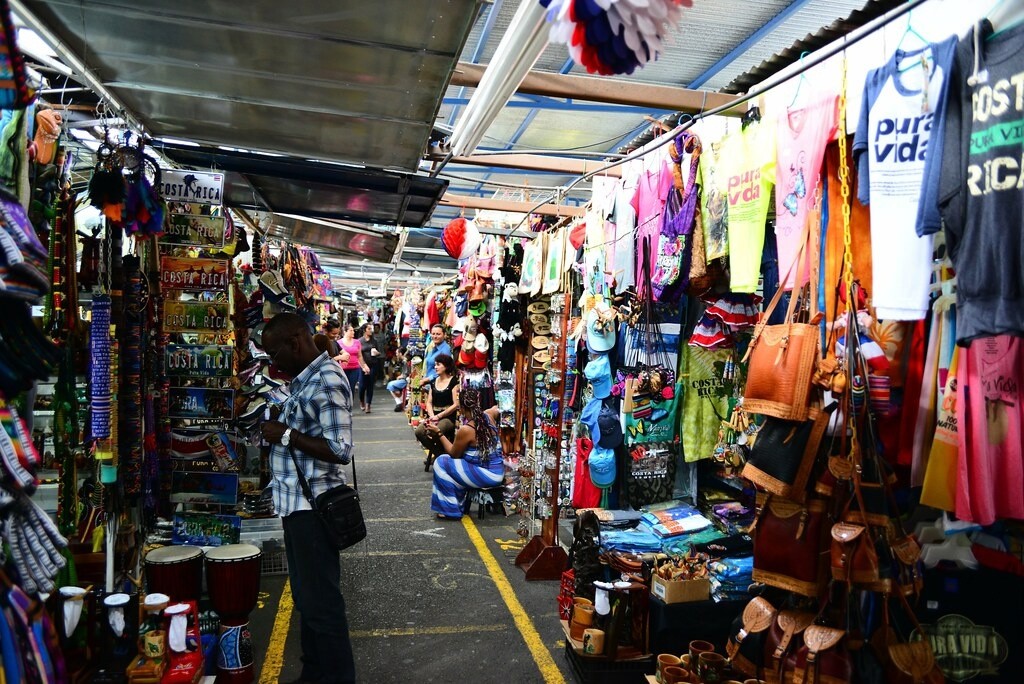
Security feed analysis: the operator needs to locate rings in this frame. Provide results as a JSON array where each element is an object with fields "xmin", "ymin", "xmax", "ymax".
[{"xmin": 260, "ymin": 431, "xmax": 262, "ymax": 434}]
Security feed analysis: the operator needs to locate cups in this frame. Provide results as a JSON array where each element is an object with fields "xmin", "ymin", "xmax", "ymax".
[
  {"xmin": 656, "ymin": 639, "xmax": 768, "ymax": 684},
  {"xmin": 205, "ymin": 431, "xmax": 239, "ymax": 471},
  {"xmin": 569, "ymin": 596, "xmax": 592, "ymax": 627},
  {"xmin": 136, "ymin": 629, "xmax": 166, "ymax": 657},
  {"xmin": 581, "ymin": 628, "xmax": 605, "ymax": 654},
  {"xmin": 411, "ymin": 416, "xmax": 420, "ymax": 430},
  {"xmin": 101, "ymin": 462, "xmax": 117, "ymax": 483},
  {"xmin": 569, "ymin": 603, "xmax": 596, "ymax": 641}
]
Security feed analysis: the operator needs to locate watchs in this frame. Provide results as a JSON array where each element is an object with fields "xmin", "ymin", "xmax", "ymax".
[{"xmin": 281, "ymin": 428, "xmax": 291, "ymax": 446}]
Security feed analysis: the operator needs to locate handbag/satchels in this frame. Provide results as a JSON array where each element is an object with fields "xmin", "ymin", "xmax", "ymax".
[
  {"xmin": 738, "ymin": 397, "xmax": 840, "ymax": 503},
  {"xmin": 315, "ymin": 484, "xmax": 369, "ymax": 551},
  {"xmin": 717, "ymin": 407, "xmax": 954, "ymax": 684},
  {"xmin": 739, "ymin": 320, "xmax": 821, "ymax": 428}
]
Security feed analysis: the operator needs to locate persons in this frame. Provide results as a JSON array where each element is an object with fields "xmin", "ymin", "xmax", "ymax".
[
  {"xmin": 336, "ymin": 325, "xmax": 369, "ymax": 395},
  {"xmin": 423, "ymin": 388, "xmax": 505, "ymax": 520},
  {"xmin": 357, "ymin": 323, "xmax": 378, "ymax": 414},
  {"xmin": 386, "ymin": 347, "xmax": 410, "ymax": 413},
  {"xmin": 321, "ymin": 319, "xmax": 350, "ymax": 366},
  {"xmin": 257, "ymin": 313, "xmax": 354, "ymax": 684},
  {"xmin": 373, "ymin": 324, "xmax": 388, "ymax": 388},
  {"xmin": 414, "ymin": 354, "xmax": 458, "ymax": 465},
  {"xmin": 418, "ymin": 324, "xmax": 452, "ymax": 393}
]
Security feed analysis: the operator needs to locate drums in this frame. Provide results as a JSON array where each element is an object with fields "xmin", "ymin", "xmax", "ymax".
[{"xmin": 143, "ymin": 544, "xmax": 262, "ymax": 683}]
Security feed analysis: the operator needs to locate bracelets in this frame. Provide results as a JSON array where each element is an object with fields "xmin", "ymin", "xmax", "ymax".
[{"xmin": 438, "ymin": 431, "xmax": 443, "ymax": 437}]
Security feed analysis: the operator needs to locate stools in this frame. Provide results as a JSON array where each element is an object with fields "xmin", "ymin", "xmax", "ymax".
[
  {"xmin": 461, "ymin": 484, "xmax": 506, "ymax": 519},
  {"xmin": 422, "ymin": 429, "xmax": 455, "ymax": 472}
]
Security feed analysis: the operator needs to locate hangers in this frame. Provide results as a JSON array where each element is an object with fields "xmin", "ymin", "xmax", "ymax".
[
  {"xmin": 786, "ymin": 51, "xmax": 823, "ymax": 112},
  {"xmin": 723, "ymin": 86, "xmax": 777, "ymax": 146},
  {"xmin": 955, "ymin": 0, "xmax": 1024, "ymax": 67},
  {"xmin": 869, "ymin": 0, "xmax": 957, "ymax": 85},
  {"xmin": 499, "ymin": 113, "xmax": 699, "ymax": 246}
]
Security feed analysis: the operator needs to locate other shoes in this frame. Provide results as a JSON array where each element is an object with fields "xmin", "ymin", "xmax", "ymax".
[
  {"xmin": 359, "ymin": 401, "xmax": 366, "ymax": 410},
  {"xmin": 436, "ymin": 512, "xmax": 460, "ymax": 519},
  {"xmin": 393, "ymin": 402, "xmax": 404, "ymax": 412},
  {"xmin": 364, "ymin": 406, "xmax": 370, "ymax": 413}
]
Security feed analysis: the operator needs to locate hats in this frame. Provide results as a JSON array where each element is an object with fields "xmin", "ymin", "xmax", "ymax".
[{"xmin": 581, "ymin": 303, "xmax": 619, "ymax": 490}]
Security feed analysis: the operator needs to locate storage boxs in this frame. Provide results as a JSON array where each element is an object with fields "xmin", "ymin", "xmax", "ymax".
[
  {"xmin": 239, "ymin": 519, "xmax": 288, "ymax": 575},
  {"xmin": 652, "ymin": 572, "xmax": 710, "ymax": 605}
]
